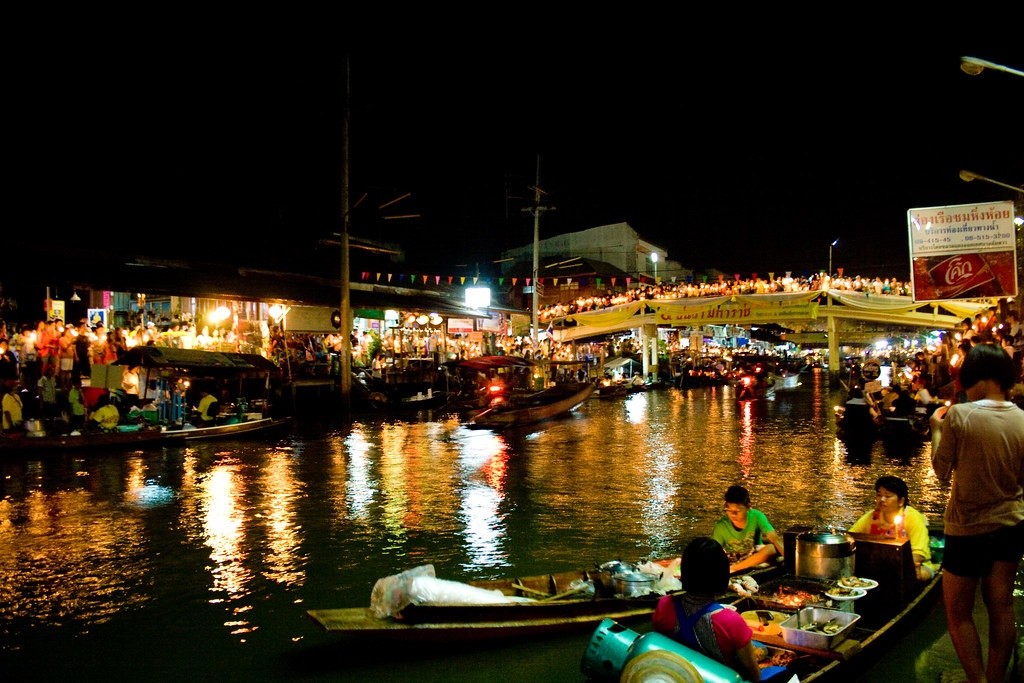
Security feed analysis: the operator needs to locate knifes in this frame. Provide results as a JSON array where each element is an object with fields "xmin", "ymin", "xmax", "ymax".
[{"xmin": 757, "ymin": 612, "xmax": 774, "ymax": 626}]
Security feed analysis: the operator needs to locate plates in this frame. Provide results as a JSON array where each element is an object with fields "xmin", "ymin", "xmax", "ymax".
[
  {"xmin": 824, "ymin": 588, "xmax": 867, "ymax": 601},
  {"xmin": 837, "ymin": 578, "xmax": 879, "ymax": 590}
]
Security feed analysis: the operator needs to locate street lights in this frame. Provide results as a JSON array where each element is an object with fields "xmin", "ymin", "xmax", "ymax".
[
  {"xmin": 650, "ymin": 251, "xmax": 659, "ymax": 282},
  {"xmin": 829, "ymin": 238, "xmax": 840, "ymax": 289}
]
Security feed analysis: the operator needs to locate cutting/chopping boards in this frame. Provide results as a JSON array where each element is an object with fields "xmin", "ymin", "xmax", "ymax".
[{"xmin": 740, "ymin": 609, "xmax": 790, "ymax": 635}]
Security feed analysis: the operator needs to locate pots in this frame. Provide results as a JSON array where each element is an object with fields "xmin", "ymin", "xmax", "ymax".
[
  {"xmin": 794, "ymin": 525, "xmax": 857, "ymax": 580},
  {"xmin": 609, "ymin": 568, "xmax": 664, "ymax": 594},
  {"xmin": 591, "ymin": 558, "xmax": 640, "ymax": 587}
]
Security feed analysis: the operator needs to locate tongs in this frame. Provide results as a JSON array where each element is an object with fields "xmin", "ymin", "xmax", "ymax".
[{"xmin": 808, "ymin": 617, "xmax": 839, "ymax": 632}]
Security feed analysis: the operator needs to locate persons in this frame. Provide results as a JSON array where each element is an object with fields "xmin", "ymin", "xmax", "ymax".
[
  {"xmin": 0, "ymin": 298, "xmax": 1024, "ymax": 434},
  {"xmin": 537, "ymin": 271, "xmax": 911, "ymax": 321},
  {"xmin": 652, "ymin": 537, "xmax": 761, "ymax": 683},
  {"xmin": 712, "ymin": 485, "xmax": 784, "ymax": 573},
  {"xmin": 929, "ymin": 342, "xmax": 1024, "ymax": 683},
  {"xmin": 850, "ymin": 476, "xmax": 935, "ymax": 581}
]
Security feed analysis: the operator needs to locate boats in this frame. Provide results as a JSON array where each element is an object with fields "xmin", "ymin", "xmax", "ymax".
[
  {"xmin": 0, "ymin": 344, "xmax": 293, "ymax": 451},
  {"xmin": 765, "ymin": 370, "xmax": 802, "ymax": 389},
  {"xmin": 458, "ymin": 351, "xmax": 600, "ymax": 426},
  {"xmin": 836, "ymin": 394, "xmax": 949, "ymax": 439},
  {"xmin": 304, "ymin": 551, "xmax": 780, "ymax": 641},
  {"xmin": 590, "ymin": 356, "xmax": 674, "ymax": 399},
  {"xmin": 726, "ymin": 523, "xmax": 944, "ymax": 683},
  {"xmin": 735, "ymin": 376, "xmax": 777, "ymax": 398}
]
